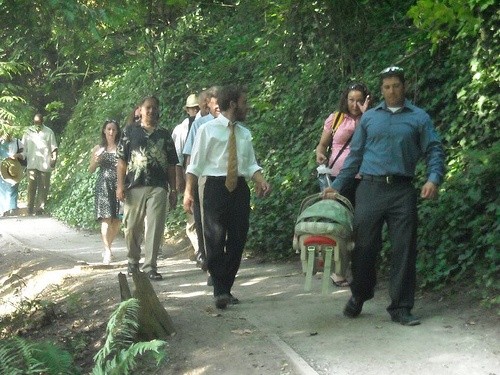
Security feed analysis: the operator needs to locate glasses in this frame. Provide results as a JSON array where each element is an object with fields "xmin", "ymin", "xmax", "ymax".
[
  {"xmin": 134, "ymin": 116, "xmax": 142, "ymax": 120},
  {"xmin": 349, "ymin": 83, "xmax": 366, "ymax": 91},
  {"xmin": 379, "ymin": 67, "xmax": 403, "ymax": 74},
  {"xmin": 106, "ymin": 120, "xmax": 117, "ymax": 123}
]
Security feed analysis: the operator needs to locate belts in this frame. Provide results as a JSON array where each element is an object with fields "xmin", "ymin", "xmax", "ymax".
[{"xmin": 363, "ymin": 176, "xmax": 411, "ymax": 184}]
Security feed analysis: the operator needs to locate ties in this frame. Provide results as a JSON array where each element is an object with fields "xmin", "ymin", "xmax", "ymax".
[{"xmin": 225, "ymin": 122, "xmax": 238, "ymax": 192}]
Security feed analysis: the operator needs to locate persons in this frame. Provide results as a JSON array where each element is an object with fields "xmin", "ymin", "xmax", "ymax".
[
  {"xmin": 316, "ymin": 66, "xmax": 446, "ymax": 327},
  {"xmin": 0, "ymin": 114, "xmax": 58, "ymax": 217},
  {"xmin": 88, "ymin": 85, "xmax": 271, "ymax": 310}
]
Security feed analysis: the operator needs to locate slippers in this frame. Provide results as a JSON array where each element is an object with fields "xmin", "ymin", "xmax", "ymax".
[{"xmin": 330, "ymin": 275, "xmax": 348, "ymax": 287}]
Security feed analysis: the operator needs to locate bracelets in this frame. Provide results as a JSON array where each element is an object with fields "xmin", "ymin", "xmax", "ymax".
[{"xmin": 170, "ymin": 189, "xmax": 177, "ymax": 193}]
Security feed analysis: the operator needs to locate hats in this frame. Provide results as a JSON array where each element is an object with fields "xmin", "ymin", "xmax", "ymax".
[
  {"xmin": 183, "ymin": 94, "xmax": 198, "ymax": 110},
  {"xmin": 0, "ymin": 157, "xmax": 23, "ymax": 184}
]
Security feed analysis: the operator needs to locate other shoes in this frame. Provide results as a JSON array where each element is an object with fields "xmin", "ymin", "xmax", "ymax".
[
  {"xmin": 215, "ymin": 295, "xmax": 227, "ymax": 308},
  {"xmin": 391, "ymin": 315, "xmax": 420, "ymax": 325},
  {"xmin": 207, "ymin": 276, "xmax": 214, "ymax": 286},
  {"xmin": 343, "ymin": 295, "xmax": 365, "ymax": 318},
  {"xmin": 128, "ymin": 262, "xmax": 139, "ymax": 273},
  {"xmin": 103, "ymin": 253, "xmax": 111, "ymax": 264},
  {"xmin": 147, "ymin": 270, "xmax": 162, "ymax": 281},
  {"xmin": 227, "ymin": 293, "xmax": 238, "ymax": 304}
]
